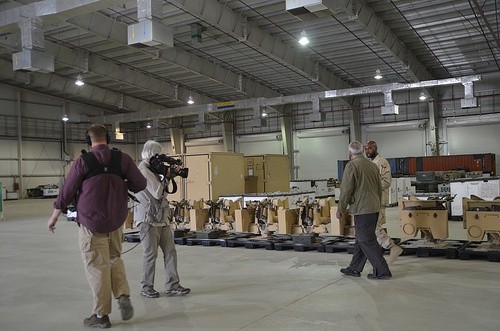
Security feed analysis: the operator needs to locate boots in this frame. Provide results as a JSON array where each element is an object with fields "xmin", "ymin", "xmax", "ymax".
[{"xmin": 388, "ymin": 245, "xmax": 403, "ymax": 264}]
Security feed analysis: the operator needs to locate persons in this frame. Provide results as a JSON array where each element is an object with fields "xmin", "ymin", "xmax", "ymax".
[
  {"xmin": 335, "ymin": 141, "xmax": 392, "ymax": 280},
  {"xmin": 364, "ymin": 140, "xmax": 403, "ymax": 264},
  {"xmin": 48, "ymin": 124, "xmax": 147, "ymax": 329},
  {"xmin": 133, "ymin": 140, "xmax": 190, "ymax": 298}
]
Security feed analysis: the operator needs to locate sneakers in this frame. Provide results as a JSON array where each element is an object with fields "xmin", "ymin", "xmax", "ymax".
[
  {"xmin": 164, "ymin": 283, "xmax": 190, "ymax": 297},
  {"xmin": 84, "ymin": 314, "xmax": 111, "ymax": 328},
  {"xmin": 340, "ymin": 267, "xmax": 361, "ymax": 277},
  {"xmin": 367, "ymin": 272, "xmax": 390, "ymax": 280},
  {"xmin": 119, "ymin": 296, "xmax": 133, "ymax": 320},
  {"xmin": 140, "ymin": 286, "xmax": 159, "ymax": 298}
]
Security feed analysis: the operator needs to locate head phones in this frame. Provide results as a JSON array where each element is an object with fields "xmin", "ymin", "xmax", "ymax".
[{"xmin": 85, "ymin": 129, "xmax": 109, "ymax": 146}]
